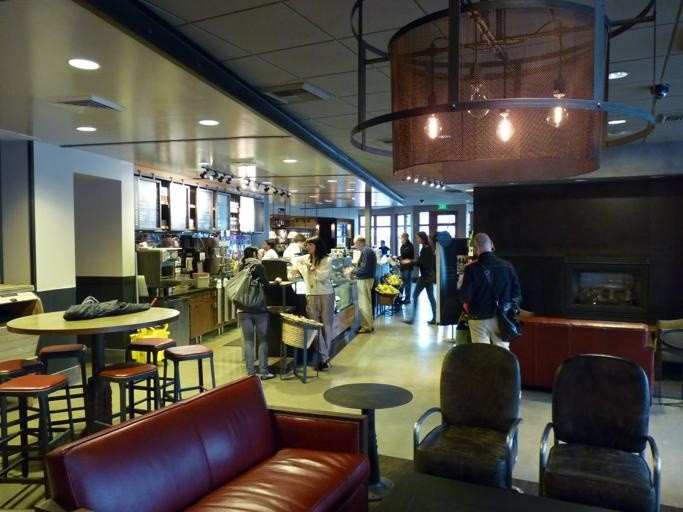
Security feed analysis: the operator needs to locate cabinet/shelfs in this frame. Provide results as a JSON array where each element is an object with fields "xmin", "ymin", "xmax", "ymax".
[
  {"xmin": 156, "ymin": 298, "xmax": 188, "ymax": 350},
  {"xmin": 245, "ymin": 278, "xmax": 361, "ymax": 364},
  {"xmin": 188, "ymin": 291, "xmax": 218, "ymax": 342},
  {"xmin": 137, "ymin": 246, "xmax": 183, "ymax": 287}
]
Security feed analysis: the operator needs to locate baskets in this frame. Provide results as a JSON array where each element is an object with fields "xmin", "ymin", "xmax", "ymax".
[
  {"xmin": 279, "ymin": 312, "xmax": 324, "ymax": 349},
  {"xmin": 375, "ymin": 286, "xmax": 398, "ymax": 305},
  {"xmin": 380, "ymin": 274, "xmax": 400, "ymax": 289}
]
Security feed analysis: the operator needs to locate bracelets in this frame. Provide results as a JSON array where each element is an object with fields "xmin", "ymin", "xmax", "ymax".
[{"xmin": 308, "ymin": 263, "xmax": 315, "ymax": 271}]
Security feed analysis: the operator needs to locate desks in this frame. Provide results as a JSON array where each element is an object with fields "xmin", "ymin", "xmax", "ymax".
[{"xmin": 5, "ymin": 305, "xmax": 181, "ymax": 439}]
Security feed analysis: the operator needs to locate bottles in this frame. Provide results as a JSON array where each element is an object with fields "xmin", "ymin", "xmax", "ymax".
[
  {"xmin": 397, "ymin": 259, "xmax": 400, "ymax": 274},
  {"xmin": 186, "ymin": 253, "xmax": 194, "ymax": 271}
]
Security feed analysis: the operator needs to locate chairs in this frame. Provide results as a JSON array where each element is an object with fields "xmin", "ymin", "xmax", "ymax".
[
  {"xmin": 536, "ymin": 351, "xmax": 663, "ymax": 511},
  {"xmin": 409, "ymin": 340, "xmax": 525, "ymax": 512}
]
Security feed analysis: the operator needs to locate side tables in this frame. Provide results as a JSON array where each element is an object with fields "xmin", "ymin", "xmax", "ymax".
[{"xmin": 323, "ymin": 382, "xmax": 413, "ymax": 505}]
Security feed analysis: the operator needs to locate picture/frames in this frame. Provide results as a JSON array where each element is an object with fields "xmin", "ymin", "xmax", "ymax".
[{"xmin": 560, "ymin": 256, "xmax": 650, "ymax": 322}]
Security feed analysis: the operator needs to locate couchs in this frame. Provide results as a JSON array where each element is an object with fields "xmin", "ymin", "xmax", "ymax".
[
  {"xmin": 508, "ymin": 314, "xmax": 657, "ymax": 407},
  {"xmin": 33, "ymin": 373, "xmax": 372, "ymax": 511}
]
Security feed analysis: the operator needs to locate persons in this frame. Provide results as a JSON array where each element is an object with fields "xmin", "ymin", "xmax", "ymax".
[
  {"xmin": 391, "ymin": 232, "xmax": 414, "ymax": 304},
  {"xmin": 346, "ymin": 235, "xmax": 377, "ymax": 334},
  {"xmin": 235, "ymin": 246, "xmax": 276, "ymax": 380},
  {"xmin": 262, "ymin": 239, "xmax": 279, "ymax": 258},
  {"xmin": 399, "ymin": 231, "xmax": 436, "ymax": 325},
  {"xmin": 290, "ymin": 235, "xmax": 333, "ymax": 371},
  {"xmin": 428, "ymin": 233, "xmax": 440, "ymax": 251},
  {"xmin": 378, "ymin": 240, "xmax": 389, "ymax": 255},
  {"xmin": 282, "ymin": 233, "xmax": 306, "ymax": 257},
  {"xmin": 459, "ymin": 232, "xmax": 520, "ymax": 352}
]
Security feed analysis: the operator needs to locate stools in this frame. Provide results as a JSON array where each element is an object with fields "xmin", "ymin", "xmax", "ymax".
[{"xmin": 1, "ymin": 336, "xmax": 216, "ymax": 481}]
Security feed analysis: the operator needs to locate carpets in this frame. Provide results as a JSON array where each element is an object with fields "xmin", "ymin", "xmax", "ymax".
[{"xmin": 321, "ymin": 451, "xmax": 682, "ymax": 512}]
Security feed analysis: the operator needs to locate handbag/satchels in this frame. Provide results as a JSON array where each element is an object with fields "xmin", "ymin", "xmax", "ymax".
[
  {"xmin": 226, "ymin": 263, "xmax": 264, "ymax": 308},
  {"xmin": 497, "ymin": 303, "xmax": 523, "ymax": 342}
]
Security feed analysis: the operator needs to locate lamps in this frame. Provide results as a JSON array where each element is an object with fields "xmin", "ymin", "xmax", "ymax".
[
  {"xmin": 464, "ymin": 14, "xmax": 490, "ymax": 119},
  {"xmin": 421, "ymin": 44, "xmax": 444, "ymax": 141},
  {"xmin": 542, "ymin": 27, "xmax": 571, "ymax": 128},
  {"xmin": 494, "ymin": 55, "xmax": 515, "ymax": 143}
]
